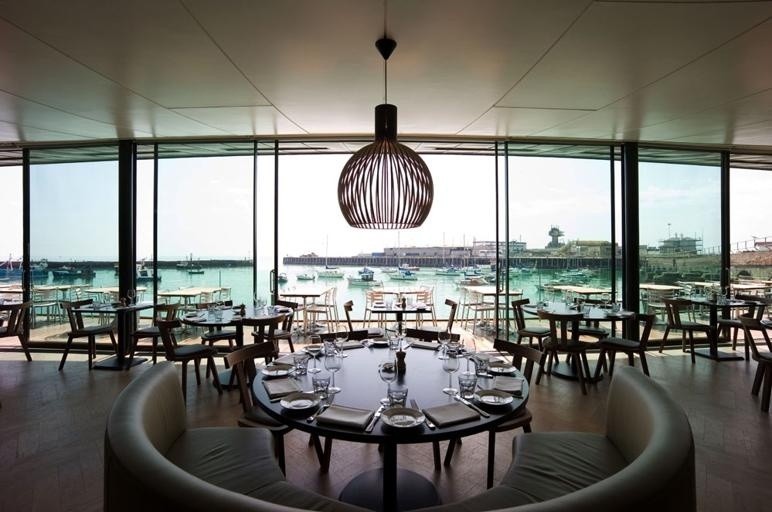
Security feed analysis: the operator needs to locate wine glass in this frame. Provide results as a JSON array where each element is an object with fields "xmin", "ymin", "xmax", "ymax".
[
  {"xmin": 443, "ymin": 349, "xmax": 460, "ymax": 394},
  {"xmin": 89, "ymin": 289, "xmax": 268, "ymax": 320},
  {"xmin": 378, "ymin": 359, "xmax": 398, "ymax": 405},
  {"xmin": 540, "ymin": 280, "xmax": 736, "ymax": 312},
  {"xmin": 263, "ymin": 322, "xmax": 517, "ymax": 375},
  {"xmin": 324, "ymin": 343, "xmax": 343, "ymax": 393}
]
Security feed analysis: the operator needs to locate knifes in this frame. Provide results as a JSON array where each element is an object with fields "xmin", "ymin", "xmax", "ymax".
[
  {"xmin": 454, "ymin": 394, "xmax": 490, "ymax": 417},
  {"xmin": 365, "ymin": 405, "xmax": 385, "ymax": 432}
]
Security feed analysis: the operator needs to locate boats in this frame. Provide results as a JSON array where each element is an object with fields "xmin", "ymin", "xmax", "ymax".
[
  {"xmin": 177, "ymin": 252, "xmax": 202, "ymax": 269},
  {"xmin": 187, "ymin": 270, "xmax": 204, "ymax": 275},
  {"xmin": 0, "ymin": 253, "xmax": 162, "ymax": 282},
  {"xmin": 348, "ymin": 261, "xmax": 599, "ymax": 295},
  {"xmin": 315, "ymin": 250, "xmax": 345, "ymax": 278},
  {"xmin": 297, "ymin": 273, "xmax": 315, "ymax": 280},
  {"xmin": 278, "ymin": 273, "xmax": 287, "ymax": 282}
]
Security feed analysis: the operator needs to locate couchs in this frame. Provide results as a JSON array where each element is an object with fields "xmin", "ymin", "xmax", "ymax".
[{"xmin": 102, "ymin": 359, "xmax": 698, "ymax": 512}]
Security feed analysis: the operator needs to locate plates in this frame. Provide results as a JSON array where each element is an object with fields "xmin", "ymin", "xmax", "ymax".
[
  {"xmin": 381, "ymin": 408, "xmax": 425, "ymax": 428},
  {"xmin": 473, "ymin": 390, "xmax": 513, "ymax": 405},
  {"xmin": 280, "ymin": 392, "xmax": 320, "ymax": 409}
]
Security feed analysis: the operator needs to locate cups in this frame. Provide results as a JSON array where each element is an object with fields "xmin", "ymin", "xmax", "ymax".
[
  {"xmin": 458, "ymin": 372, "xmax": 478, "ymax": 400},
  {"xmin": 312, "ymin": 376, "xmax": 330, "ymax": 399},
  {"xmin": 387, "ymin": 385, "xmax": 408, "ymax": 407}
]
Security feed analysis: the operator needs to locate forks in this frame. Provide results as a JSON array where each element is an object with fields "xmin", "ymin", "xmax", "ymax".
[
  {"xmin": 306, "ymin": 392, "xmax": 334, "ymax": 421},
  {"xmin": 409, "ymin": 399, "xmax": 436, "ymax": 429}
]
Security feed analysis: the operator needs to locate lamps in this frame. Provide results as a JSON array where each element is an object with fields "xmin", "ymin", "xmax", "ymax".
[{"xmin": 337, "ymin": 38, "xmax": 434, "ymax": 231}]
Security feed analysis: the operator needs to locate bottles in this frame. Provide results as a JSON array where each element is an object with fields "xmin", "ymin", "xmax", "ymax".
[{"xmin": 401, "ymin": 297, "xmax": 407, "ymax": 309}]
